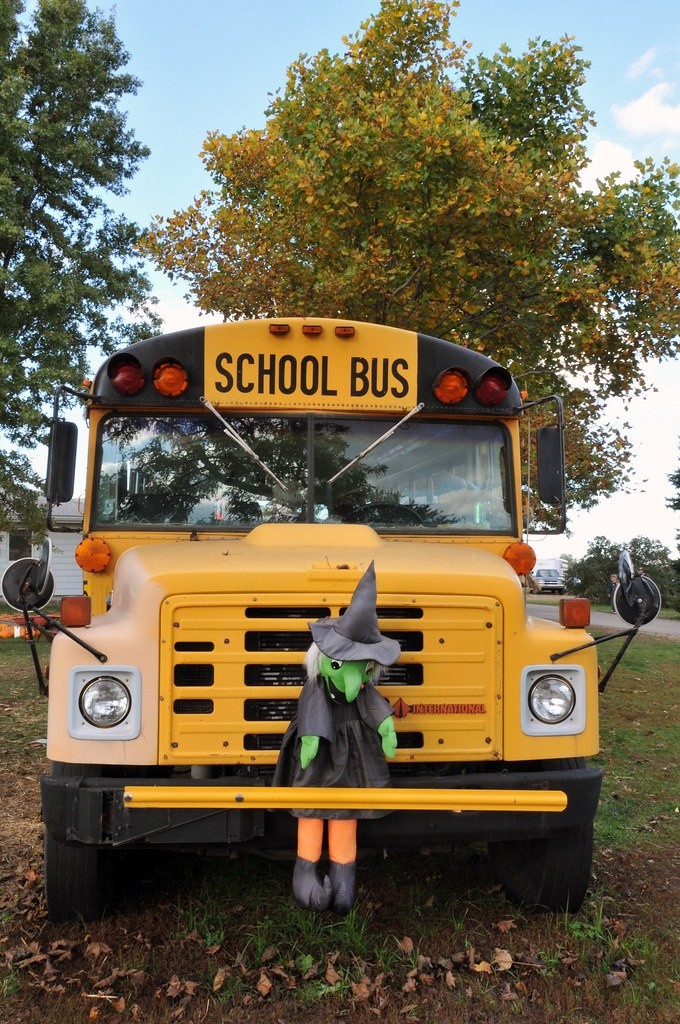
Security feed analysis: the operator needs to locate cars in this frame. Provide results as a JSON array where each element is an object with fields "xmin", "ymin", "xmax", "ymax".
[{"xmin": 529, "ymin": 566, "xmax": 565, "ymax": 595}]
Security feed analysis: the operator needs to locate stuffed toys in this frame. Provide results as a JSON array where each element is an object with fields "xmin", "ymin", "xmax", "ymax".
[{"xmin": 269, "ymin": 558, "xmax": 402, "ymax": 911}]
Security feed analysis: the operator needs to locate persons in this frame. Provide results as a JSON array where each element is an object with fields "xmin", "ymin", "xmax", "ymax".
[{"xmin": 607, "ymin": 574, "xmax": 618, "ymax": 615}]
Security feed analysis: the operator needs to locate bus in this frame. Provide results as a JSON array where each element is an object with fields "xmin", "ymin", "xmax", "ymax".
[{"xmin": 0, "ymin": 315, "xmax": 662, "ymax": 915}]
